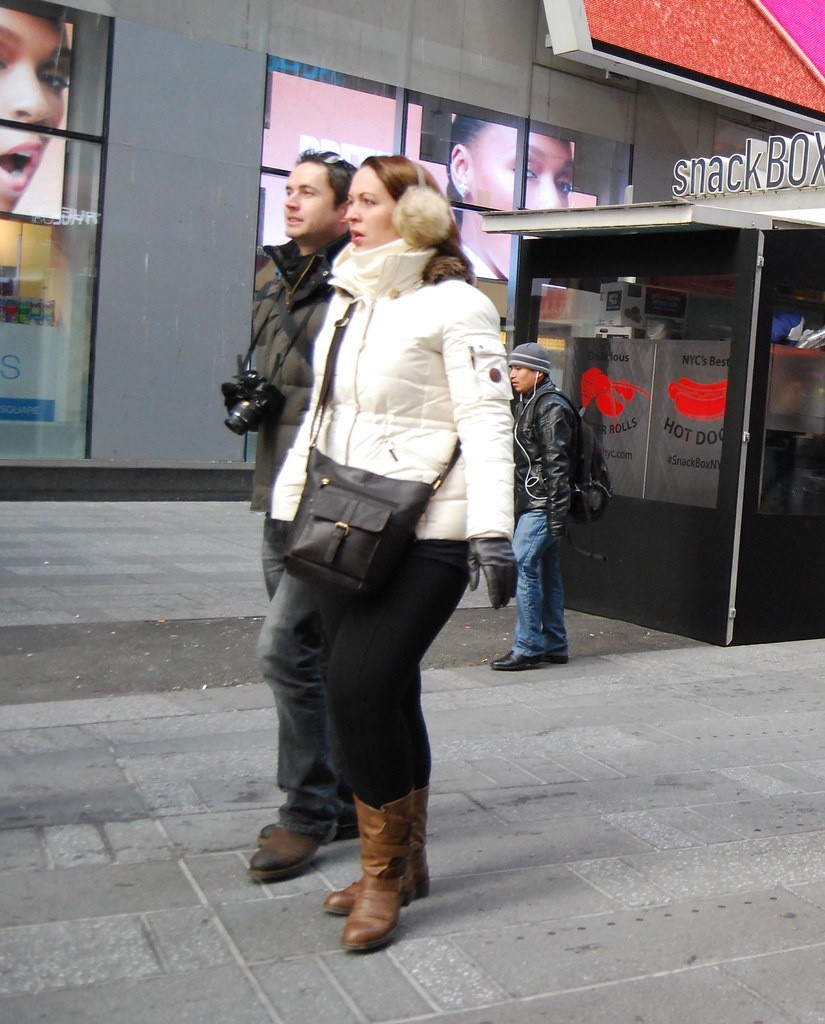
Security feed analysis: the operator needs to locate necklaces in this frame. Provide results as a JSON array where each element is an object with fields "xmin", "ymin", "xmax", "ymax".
[{"xmin": 284, "ymin": 255, "xmax": 315, "ymax": 309}]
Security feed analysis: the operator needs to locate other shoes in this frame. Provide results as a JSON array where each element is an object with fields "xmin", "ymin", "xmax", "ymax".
[
  {"xmin": 246, "ymin": 826, "xmax": 320, "ymax": 881},
  {"xmin": 256, "ymin": 823, "xmax": 360, "ymax": 846}
]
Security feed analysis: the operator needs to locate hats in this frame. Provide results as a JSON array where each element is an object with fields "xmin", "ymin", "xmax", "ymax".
[{"xmin": 508, "ymin": 342, "xmax": 550, "ymax": 375}]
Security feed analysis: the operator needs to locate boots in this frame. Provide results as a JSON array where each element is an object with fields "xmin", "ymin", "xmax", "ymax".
[
  {"xmin": 340, "ymin": 786, "xmax": 416, "ymax": 950},
  {"xmin": 323, "ymin": 782, "xmax": 431, "ymax": 915}
]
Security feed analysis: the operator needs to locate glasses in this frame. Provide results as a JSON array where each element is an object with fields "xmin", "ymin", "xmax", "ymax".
[{"xmin": 310, "ymin": 152, "xmax": 351, "ymax": 177}]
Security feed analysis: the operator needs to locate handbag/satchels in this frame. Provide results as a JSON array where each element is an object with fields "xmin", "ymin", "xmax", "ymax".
[{"xmin": 281, "ymin": 437, "xmax": 447, "ymax": 602}]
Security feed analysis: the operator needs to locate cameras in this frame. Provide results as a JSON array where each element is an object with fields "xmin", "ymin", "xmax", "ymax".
[{"xmin": 221, "ymin": 369, "xmax": 286, "ymax": 436}]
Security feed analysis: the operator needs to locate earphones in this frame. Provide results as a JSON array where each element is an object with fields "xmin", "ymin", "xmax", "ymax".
[{"xmin": 536, "ymin": 371, "xmax": 539, "ymax": 378}]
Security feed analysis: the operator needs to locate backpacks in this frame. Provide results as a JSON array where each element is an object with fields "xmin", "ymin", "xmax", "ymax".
[{"xmin": 528, "ymin": 389, "xmax": 613, "ymax": 525}]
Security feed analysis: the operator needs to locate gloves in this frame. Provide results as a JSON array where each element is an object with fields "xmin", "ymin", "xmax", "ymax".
[
  {"xmin": 548, "ymin": 520, "xmax": 573, "ymax": 554},
  {"xmin": 467, "ymin": 536, "xmax": 519, "ymax": 610},
  {"xmin": 221, "ymin": 369, "xmax": 263, "ymax": 413}
]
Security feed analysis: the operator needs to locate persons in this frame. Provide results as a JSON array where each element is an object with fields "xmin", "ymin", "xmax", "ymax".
[
  {"xmin": 445, "ymin": 114, "xmax": 574, "ymax": 282},
  {"xmin": 269, "ymin": 154, "xmax": 519, "ymax": 954},
  {"xmin": 0, "ymin": 6, "xmax": 73, "ymax": 214},
  {"xmin": 760, "ymin": 335, "xmax": 806, "ymax": 516},
  {"xmin": 490, "ymin": 343, "xmax": 580, "ymax": 671},
  {"xmin": 222, "ymin": 150, "xmax": 360, "ymax": 882}
]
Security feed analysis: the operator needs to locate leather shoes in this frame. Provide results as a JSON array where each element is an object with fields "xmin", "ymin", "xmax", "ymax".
[
  {"xmin": 544, "ymin": 654, "xmax": 571, "ymax": 664},
  {"xmin": 491, "ymin": 650, "xmax": 545, "ymax": 670}
]
